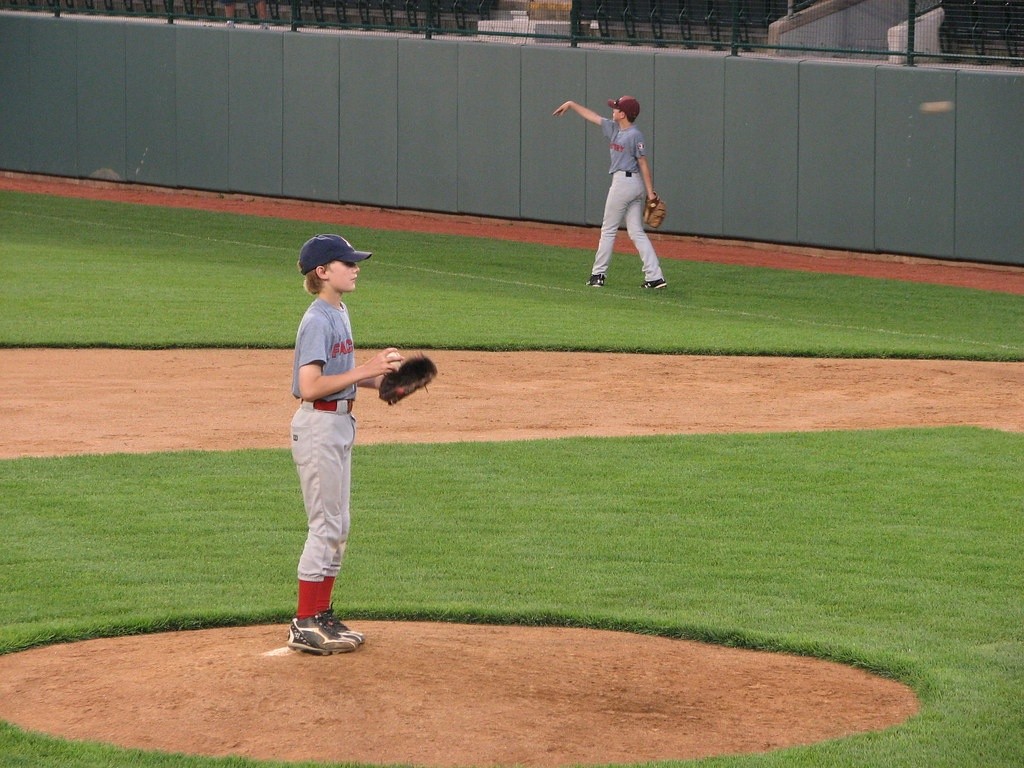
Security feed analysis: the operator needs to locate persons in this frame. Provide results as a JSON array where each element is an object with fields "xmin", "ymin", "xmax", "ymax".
[
  {"xmin": 288, "ymin": 233, "xmax": 415, "ymax": 657},
  {"xmin": 550, "ymin": 94, "xmax": 667, "ymax": 290}
]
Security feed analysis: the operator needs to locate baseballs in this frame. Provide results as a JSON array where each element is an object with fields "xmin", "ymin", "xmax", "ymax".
[{"xmin": 386, "ymin": 352, "xmax": 402, "ymax": 368}]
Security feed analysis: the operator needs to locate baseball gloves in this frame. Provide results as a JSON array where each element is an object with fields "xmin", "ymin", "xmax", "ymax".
[
  {"xmin": 643, "ymin": 191, "xmax": 667, "ymax": 228},
  {"xmin": 378, "ymin": 351, "xmax": 437, "ymax": 407}
]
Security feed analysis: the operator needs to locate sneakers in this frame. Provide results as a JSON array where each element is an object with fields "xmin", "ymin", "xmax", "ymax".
[
  {"xmin": 322, "ymin": 609, "xmax": 365, "ymax": 644},
  {"xmin": 287, "ymin": 617, "xmax": 357, "ymax": 656},
  {"xmin": 585, "ymin": 275, "xmax": 605, "ymax": 287},
  {"xmin": 641, "ymin": 280, "xmax": 667, "ymax": 288}
]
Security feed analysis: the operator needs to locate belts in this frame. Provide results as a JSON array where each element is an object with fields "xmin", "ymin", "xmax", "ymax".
[{"xmin": 301, "ymin": 398, "xmax": 352, "ymax": 413}]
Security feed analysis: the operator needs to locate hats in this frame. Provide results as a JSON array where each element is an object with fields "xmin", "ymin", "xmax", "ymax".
[
  {"xmin": 300, "ymin": 234, "xmax": 373, "ymax": 274},
  {"xmin": 608, "ymin": 96, "xmax": 640, "ymax": 118}
]
